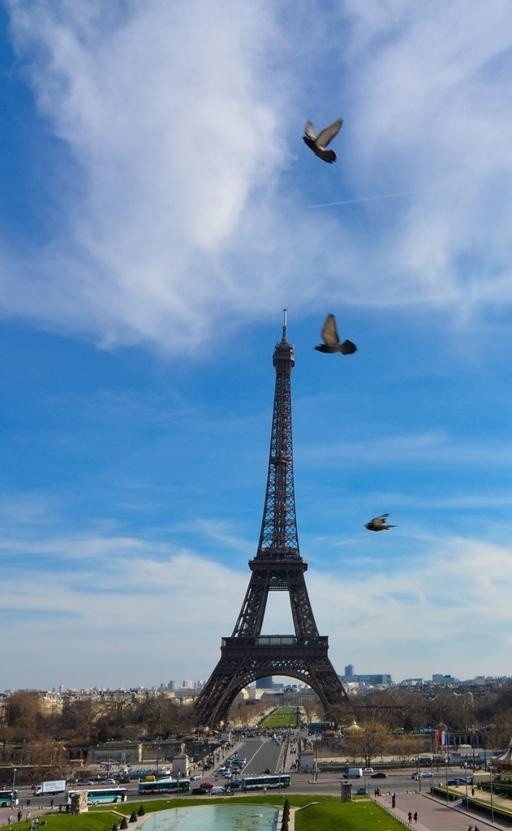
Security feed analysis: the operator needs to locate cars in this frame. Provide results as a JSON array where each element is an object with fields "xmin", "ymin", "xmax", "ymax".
[
  {"xmin": 217, "ymin": 752, "xmax": 246, "ymax": 778},
  {"xmin": 77, "ymin": 779, "xmax": 94, "ymax": 786},
  {"xmin": 357, "ymin": 787, "xmax": 368, "ymax": 794},
  {"xmin": 192, "ymin": 782, "xmax": 225, "ymax": 794},
  {"xmin": 371, "ymin": 773, "xmax": 385, "ymax": 777},
  {"xmin": 101, "ymin": 778, "xmax": 115, "ymax": 784},
  {"xmin": 363, "ymin": 768, "xmax": 373, "ymax": 773},
  {"xmin": 191, "ymin": 775, "xmax": 201, "ymax": 781},
  {"xmin": 419, "ymin": 771, "xmax": 432, "ymax": 776},
  {"xmin": 232, "ymin": 727, "xmax": 291, "ymax": 745}
]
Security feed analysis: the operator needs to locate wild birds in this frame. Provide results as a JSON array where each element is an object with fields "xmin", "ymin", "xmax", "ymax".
[
  {"xmin": 301, "ymin": 116, "xmax": 344, "ymax": 163},
  {"xmin": 364, "ymin": 513, "xmax": 398, "ymax": 531},
  {"xmin": 313, "ymin": 314, "xmax": 357, "ymax": 355}
]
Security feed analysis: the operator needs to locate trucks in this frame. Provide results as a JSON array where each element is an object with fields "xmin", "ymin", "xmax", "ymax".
[
  {"xmin": 343, "ymin": 767, "xmax": 362, "ymax": 778},
  {"xmin": 447, "ymin": 753, "xmax": 482, "ymax": 766},
  {"xmin": 33, "ymin": 779, "xmax": 65, "ymax": 796},
  {"xmin": 409, "ymin": 753, "xmax": 434, "ymax": 764}
]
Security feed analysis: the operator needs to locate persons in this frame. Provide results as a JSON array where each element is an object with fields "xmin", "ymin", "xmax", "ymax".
[
  {"xmin": 375, "ymin": 786, "xmax": 382, "ymax": 797},
  {"xmin": 263, "ymin": 786, "xmax": 266, "ymax": 794},
  {"xmin": 414, "ymin": 811, "xmax": 418, "ymax": 823},
  {"xmin": 468, "ymin": 825, "xmax": 473, "ymax": 831},
  {"xmin": 110, "ymin": 764, "xmax": 133, "ymax": 784},
  {"xmin": 0, "ymin": 775, "xmax": 47, "ymax": 831},
  {"xmin": 188, "ymin": 740, "xmax": 235, "ymax": 775},
  {"xmin": 287, "ymin": 737, "xmax": 300, "ymax": 770},
  {"xmin": 475, "ymin": 826, "xmax": 479, "ymax": 831},
  {"xmin": 408, "ymin": 811, "xmax": 412, "ymax": 823},
  {"xmin": 460, "ymin": 756, "xmax": 482, "ymax": 771}
]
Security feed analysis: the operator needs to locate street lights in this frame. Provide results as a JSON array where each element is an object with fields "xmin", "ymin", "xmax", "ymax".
[
  {"xmin": 445, "ymin": 758, "xmax": 449, "ymax": 802},
  {"xmin": 12, "ymin": 767, "xmax": 16, "ymax": 811},
  {"xmin": 464, "ymin": 761, "xmax": 468, "ymax": 809},
  {"xmin": 177, "ymin": 768, "xmax": 181, "ymax": 799}
]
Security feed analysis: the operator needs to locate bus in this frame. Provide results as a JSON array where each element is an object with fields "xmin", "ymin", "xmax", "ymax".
[
  {"xmin": 65, "ymin": 787, "xmax": 128, "ymax": 805},
  {"xmin": 138, "ymin": 779, "xmax": 190, "ymax": 794},
  {"xmin": 242, "ymin": 774, "xmax": 291, "ymax": 791},
  {"xmin": 0, "ymin": 789, "xmax": 20, "ymax": 807}
]
